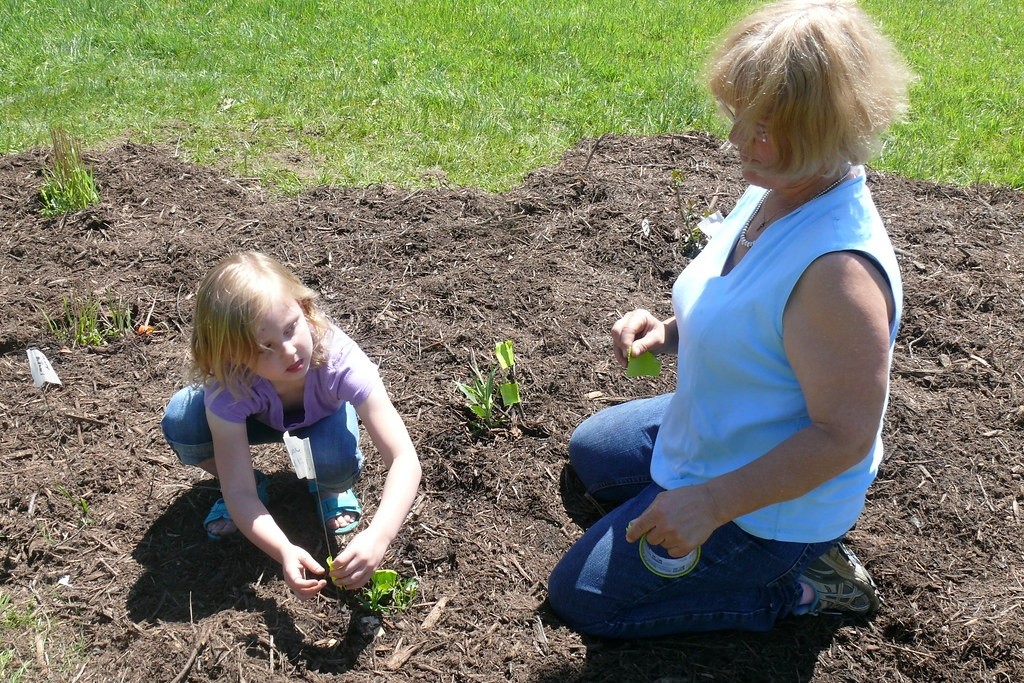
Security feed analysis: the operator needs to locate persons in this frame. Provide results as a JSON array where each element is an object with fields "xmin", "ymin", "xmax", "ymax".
[
  {"xmin": 548, "ymin": 0, "xmax": 904, "ymax": 640},
  {"xmin": 161, "ymin": 251, "xmax": 422, "ymax": 594}
]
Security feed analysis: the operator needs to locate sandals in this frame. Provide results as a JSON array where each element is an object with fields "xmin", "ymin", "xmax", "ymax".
[
  {"xmin": 308, "ymin": 479, "xmax": 362, "ymax": 535},
  {"xmin": 204, "ymin": 468, "xmax": 271, "ymax": 542}
]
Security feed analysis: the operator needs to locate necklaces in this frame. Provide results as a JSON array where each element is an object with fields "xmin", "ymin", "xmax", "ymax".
[{"xmin": 740, "ymin": 163, "xmax": 852, "ymax": 248}]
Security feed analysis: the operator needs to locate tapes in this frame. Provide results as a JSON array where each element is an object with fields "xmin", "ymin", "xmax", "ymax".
[{"xmin": 638, "ymin": 527, "xmax": 702, "ymax": 579}]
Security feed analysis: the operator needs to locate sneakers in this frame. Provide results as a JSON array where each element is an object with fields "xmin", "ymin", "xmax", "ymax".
[{"xmin": 793, "ymin": 541, "xmax": 880, "ymax": 620}]
